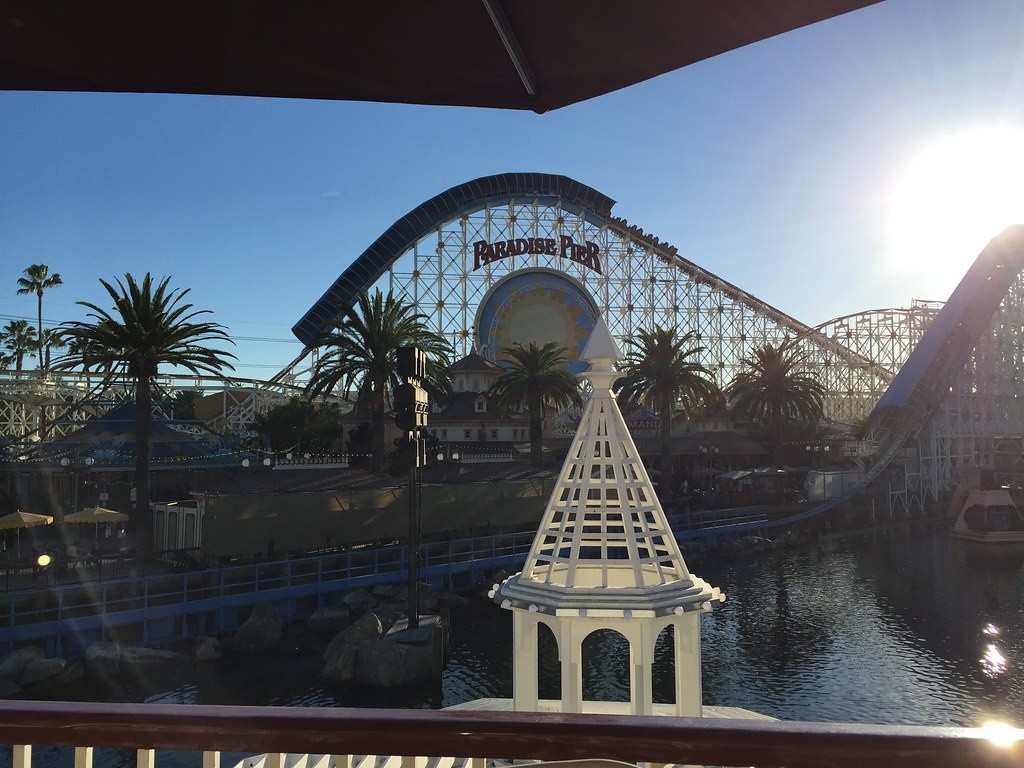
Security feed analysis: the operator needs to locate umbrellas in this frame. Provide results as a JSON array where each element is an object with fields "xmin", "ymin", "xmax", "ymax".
[
  {"xmin": 64, "ymin": 505, "xmax": 129, "ymax": 538},
  {"xmin": 0, "ymin": 509, "xmax": 53, "ymax": 560}
]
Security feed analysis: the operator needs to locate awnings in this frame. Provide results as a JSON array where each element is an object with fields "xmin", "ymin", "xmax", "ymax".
[{"xmin": 716, "ymin": 470, "xmax": 751, "ymax": 480}]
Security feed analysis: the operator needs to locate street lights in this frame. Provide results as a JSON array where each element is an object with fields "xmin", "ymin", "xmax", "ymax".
[
  {"xmin": 61, "ymin": 456, "xmax": 94, "ymax": 513},
  {"xmin": 436, "ymin": 452, "xmax": 458, "ymax": 483},
  {"xmin": 807, "ymin": 445, "xmax": 829, "ymax": 470},
  {"xmin": 241, "ymin": 457, "xmax": 271, "ymax": 493},
  {"xmin": 698, "ymin": 445, "xmax": 719, "ymax": 495}
]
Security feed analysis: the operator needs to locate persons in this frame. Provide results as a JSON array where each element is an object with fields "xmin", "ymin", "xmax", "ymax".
[
  {"xmin": 67, "ymin": 540, "xmax": 103, "ymax": 568},
  {"xmin": 105, "ymin": 524, "xmax": 112, "ymax": 538}
]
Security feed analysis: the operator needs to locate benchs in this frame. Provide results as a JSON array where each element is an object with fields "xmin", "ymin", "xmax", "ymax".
[{"xmin": 1, "ymin": 554, "xmax": 126, "ymax": 574}]
